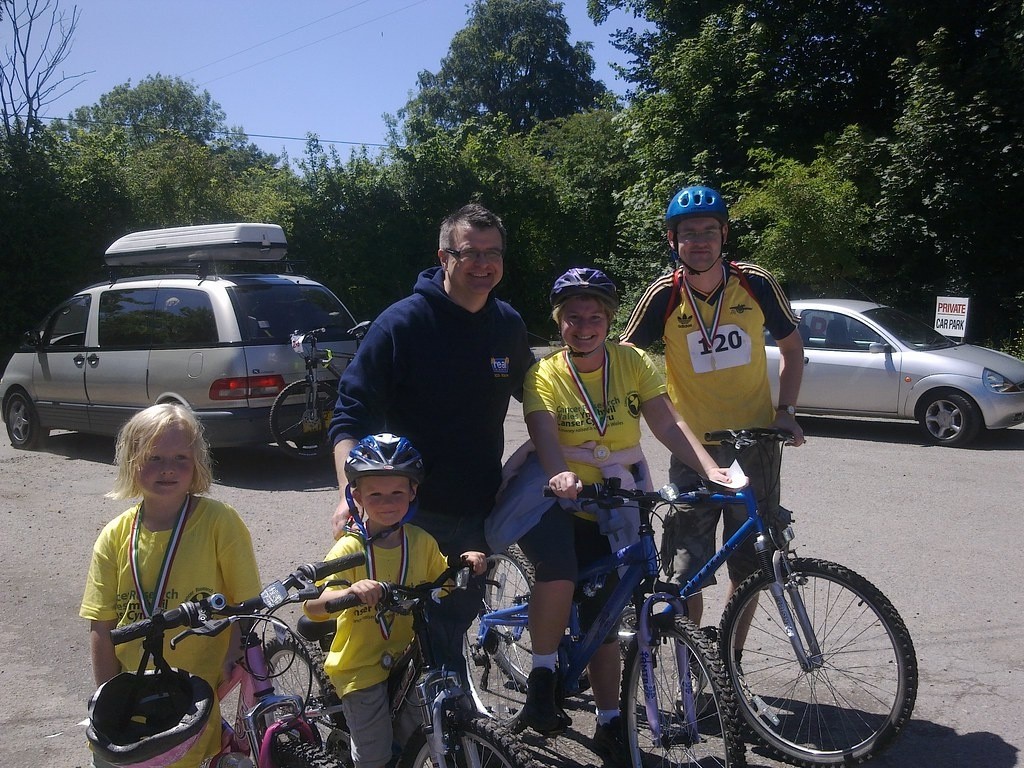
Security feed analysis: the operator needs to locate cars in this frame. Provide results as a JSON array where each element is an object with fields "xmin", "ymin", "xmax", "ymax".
[{"xmin": 764, "ymin": 298, "xmax": 1024, "ymax": 447}]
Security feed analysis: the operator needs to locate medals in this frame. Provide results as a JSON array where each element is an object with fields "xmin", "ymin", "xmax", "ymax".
[
  {"xmin": 139, "ymin": 644, "xmax": 154, "ymax": 662},
  {"xmin": 380, "ymin": 652, "xmax": 394, "ymax": 669},
  {"xmin": 710, "ymin": 352, "xmax": 717, "ymax": 372},
  {"xmin": 593, "ymin": 445, "xmax": 610, "ymax": 461}
]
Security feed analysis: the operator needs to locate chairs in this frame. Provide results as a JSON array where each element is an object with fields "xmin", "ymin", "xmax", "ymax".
[
  {"xmin": 825, "ymin": 320, "xmax": 859, "ymax": 348},
  {"xmin": 798, "ymin": 324, "xmax": 811, "ymax": 343}
]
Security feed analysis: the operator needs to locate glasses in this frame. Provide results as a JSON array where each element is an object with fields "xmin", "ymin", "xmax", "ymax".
[
  {"xmin": 445, "ymin": 247, "xmax": 503, "ymax": 262},
  {"xmin": 676, "ymin": 224, "xmax": 723, "ymax": 243}
]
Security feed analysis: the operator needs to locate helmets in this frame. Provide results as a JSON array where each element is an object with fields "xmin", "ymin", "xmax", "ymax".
[
  {"xmin": 664, "ymin": 188, "xmax": 729, "ymax": 236},
  {"xmin": 550, "ymin": 268, "xmax": 620, "ymax": 312},
  {"xmin": 343, "ymin": 433, "xmax": 423, "ymax": 488},
  {"xmin": 86, "ymin": 668, "xmax": 214, "ymax": 768}
]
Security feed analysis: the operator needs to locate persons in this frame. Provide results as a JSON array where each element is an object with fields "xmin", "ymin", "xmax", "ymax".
[
  {"xmin": 78, "ymin": 400, "xmax": 265, "ymax": 768},
  {"xmin": 329, "ymin": 205, "xmax": 537, "ymax": 688},
  {"xmin": 302, "ymin": 432, "xmax": 487, "ymax": 768},
  {"xmin": 618, "ymin": 185, "xmax": 804, "ymax": 717},
  {"xmin": 514, "ymin": 268, "xmax": 749, "ymax": 768}
]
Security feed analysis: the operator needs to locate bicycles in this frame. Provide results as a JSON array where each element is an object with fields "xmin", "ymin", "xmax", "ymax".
[
  {"xmin": 109, "ymin": 552, "xmax": 367, "ymax": 768},
  {"xmin": 268, "ymin": 320, "xmax": 373, "ymax": 464},
  {"xmin": 461, "ymin": 474, "xmax": 755, "ymax": 767},
  {"xmin": 262, "ymin": 557, "xmax": 541, "ymax": 768},
  {"xmin": 618, "ymin": 426, "xmax": 921, "ymax": 767}
]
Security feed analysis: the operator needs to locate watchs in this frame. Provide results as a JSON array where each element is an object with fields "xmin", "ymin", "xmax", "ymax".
[{"xmin": 776, "ymin": 404, "xmax": 795, "ymax": 415}]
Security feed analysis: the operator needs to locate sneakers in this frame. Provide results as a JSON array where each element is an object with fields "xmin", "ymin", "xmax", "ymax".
[
  {"xmin": 671, "ymin": 660, "xmax": 707, "ymax": 714},
  {"xmin": 735, "ymin": 661, "xmax": 754, "ymax": 707},
  {"xmin": 593, "ymin": 713, "xmax": 645, "ymax": 768},
  {"xmin": 523, "ymin": 667, "xmax": 568, "ymax": 732}
]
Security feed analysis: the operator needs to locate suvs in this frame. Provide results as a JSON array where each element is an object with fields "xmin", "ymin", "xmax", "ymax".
[{"xmin": 0, "ymin": 224, "xmax": 365, "ymax": 452}]
